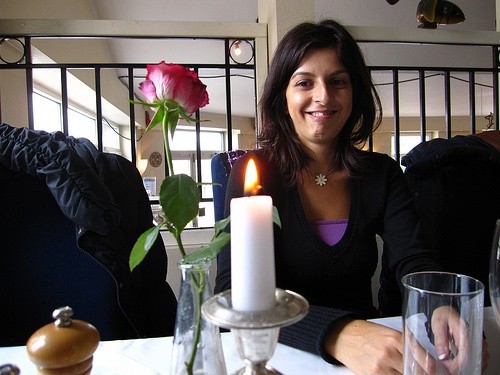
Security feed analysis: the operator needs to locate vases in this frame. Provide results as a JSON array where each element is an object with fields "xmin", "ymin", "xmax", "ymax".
[{"xmin": 169, "ymin": 255, "xmax": 227, "ymax": 375}]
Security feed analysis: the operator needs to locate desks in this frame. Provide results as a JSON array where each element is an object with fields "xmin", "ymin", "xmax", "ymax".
[{"xmin": 0, "ymin": 307, "xmax": 500, "ymax": 375}]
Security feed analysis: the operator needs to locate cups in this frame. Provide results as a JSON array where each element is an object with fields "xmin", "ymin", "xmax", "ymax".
[
  {"xmin": 489, "ymin": 219, "xmax": 500, "ymax": 327},
  {"xmin": 401, "ymin": 270, "xmax": 484, "ymax": 375}
]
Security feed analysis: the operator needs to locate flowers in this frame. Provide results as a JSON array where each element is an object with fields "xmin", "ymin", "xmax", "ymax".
[{"xmin": 127, "ymin": 61, "xmax": 281, "ymax": 375}]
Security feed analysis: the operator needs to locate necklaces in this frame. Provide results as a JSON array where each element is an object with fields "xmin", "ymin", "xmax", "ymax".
[{"xmin": 305, "ymin": 164, "xmax": 338, "ymax": 186}]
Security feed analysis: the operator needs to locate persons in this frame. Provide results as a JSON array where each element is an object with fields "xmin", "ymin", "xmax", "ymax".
[
  {"xmin": 379, "ymin": 130, "xmax": 500, "ymax": 319},
  {"xmin": 215, "ymin": 19, "xmax": 488, "ymax": 375}
]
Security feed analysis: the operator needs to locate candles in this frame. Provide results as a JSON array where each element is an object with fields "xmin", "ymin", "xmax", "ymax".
[{"xmin": 229, "ymin": 158, "xmax": 276, "ymax": 311}]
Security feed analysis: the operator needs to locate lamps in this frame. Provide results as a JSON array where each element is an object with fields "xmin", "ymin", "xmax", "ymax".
[{"xmin": 234, "ymin": 40, "xmax": 242, "ymax": 55}]
